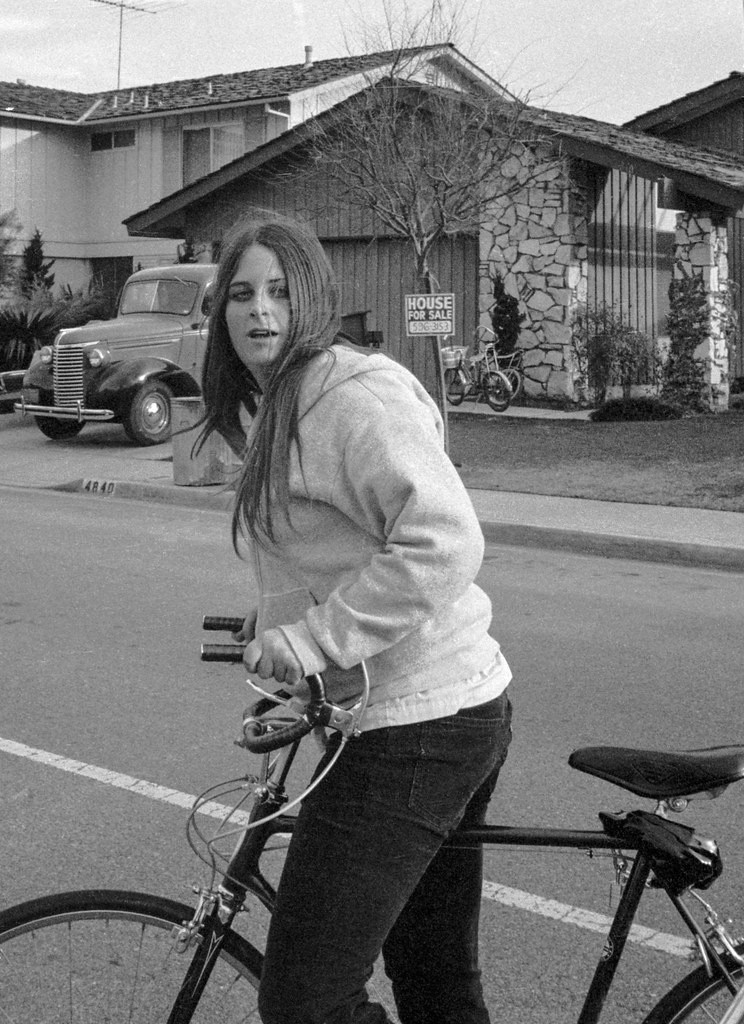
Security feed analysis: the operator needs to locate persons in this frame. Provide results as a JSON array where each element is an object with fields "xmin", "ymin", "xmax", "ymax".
[{"xmin": 162, "ymin": 216, "xmax": 518, "ymax": 1024}]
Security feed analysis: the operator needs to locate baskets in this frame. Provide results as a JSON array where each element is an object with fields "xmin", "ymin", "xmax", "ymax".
[{"xmin": 441, "ymin": 345, "xmax": 469, "ymax": 368}]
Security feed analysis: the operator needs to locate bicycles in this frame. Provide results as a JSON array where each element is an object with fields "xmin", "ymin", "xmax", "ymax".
[
  {"xmin": 0, "ymin": 610, "xmax": 743, "ymax": 1024},
  {"xmin": 436, "ymin": 322, "xmax": 523, "ymax": 412}
]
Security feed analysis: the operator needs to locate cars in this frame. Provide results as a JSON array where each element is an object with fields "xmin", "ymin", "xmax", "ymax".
[{"xmin": 17, "ymin": 261, "xmax": 229, "ymax": 448}]
